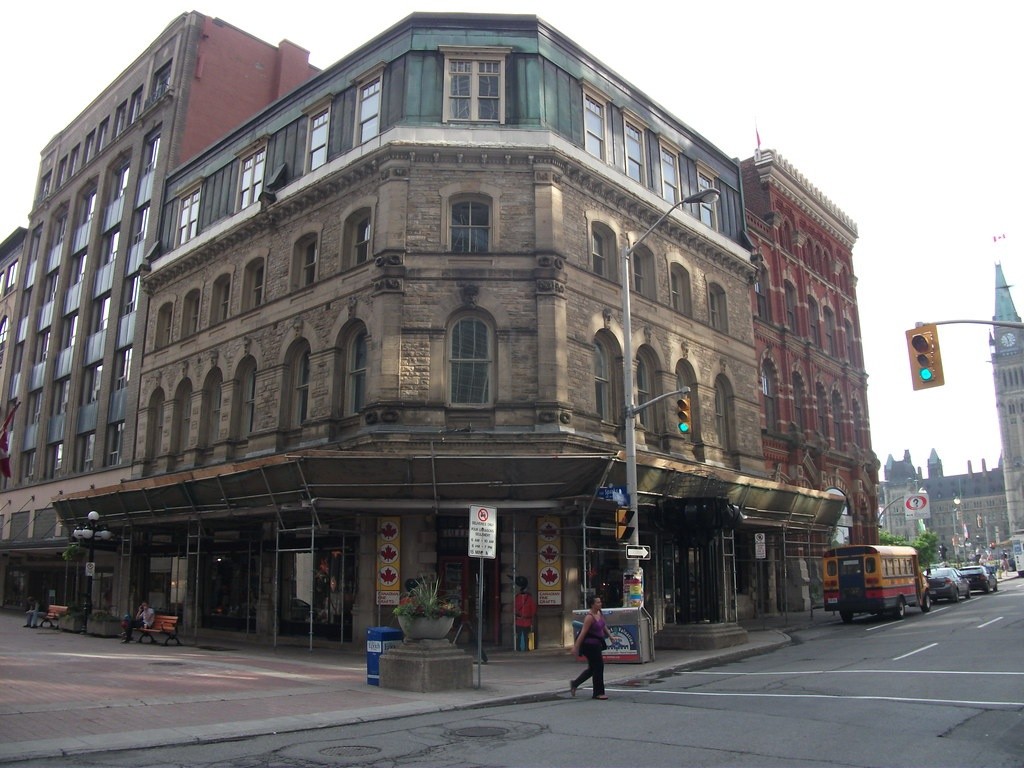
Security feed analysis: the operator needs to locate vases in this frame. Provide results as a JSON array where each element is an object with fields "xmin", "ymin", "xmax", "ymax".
[{"xmin": 397, "ymin": 615, "xmax": 454, "ymax": 639}]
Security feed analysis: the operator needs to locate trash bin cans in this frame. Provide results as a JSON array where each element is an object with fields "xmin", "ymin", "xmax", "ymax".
[{"xmin": 365, "ymin": 626, "xmax": 403, "ymax": 687}]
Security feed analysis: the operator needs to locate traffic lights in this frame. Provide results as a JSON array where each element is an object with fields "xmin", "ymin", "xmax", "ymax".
[
  {"xmin": 912, "ymin": 332, "xmax": 935, "ymax": 382},
  {"xmin": 618, "ymin": 510, "xmax": 636, "ymax": 540},
  {"xmin": 676, "ymin": 398, "xmax": 690, "ymax": 433}
]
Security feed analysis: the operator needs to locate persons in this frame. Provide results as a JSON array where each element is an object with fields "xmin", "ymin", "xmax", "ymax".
[
  {"xmin": 80, "ymin": 595, "xmax": 91, "ymax": 635},
  {"xmin": 938, "ymin": 543, "xmax": 948, "ymax": 562},
  {"xmin": 22, "ymin": 597, "xmax": 48, "ymax": 629},
  {"xmin": 120, "ymin": 600, "xmax": 154, "ymax": 644},
  {"xmin": 1000, "ymin": 549, "xmax": 1010, "ymax": 577},
  {"xmin": 570, "ymin": 595, "xmax": 618, "ymax": 700},
  {"xmin": 512, "ymin": 587, "xmax": 536, "ymax": 652}
]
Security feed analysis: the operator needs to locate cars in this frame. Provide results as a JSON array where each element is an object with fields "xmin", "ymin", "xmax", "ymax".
[
  {"xmin": 957, "ymin": 566, "xmax": 998, "ymax": 593},
  {"xmin": 922, "ymin": 568, "xmax": 971, "ymax": 603}
]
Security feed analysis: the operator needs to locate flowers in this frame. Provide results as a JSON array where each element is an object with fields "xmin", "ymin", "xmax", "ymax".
[{"xmin": 388, "ymin": 573, "xmax": 468, "ymax": 630}]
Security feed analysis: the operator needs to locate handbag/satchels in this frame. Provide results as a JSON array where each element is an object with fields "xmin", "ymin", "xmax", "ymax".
[
  {"xmin": 600, "ymin": 637, "xmax": 608, "ymax": 651},
  {"xmin": 527, "ymin": 626, "xmax": 535, "ymax": 651},
  {"xmin": 513, "ymin": 611, "xmax": 522, "ymax": 619}
]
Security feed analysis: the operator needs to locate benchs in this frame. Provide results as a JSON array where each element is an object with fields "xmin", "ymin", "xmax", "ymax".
[
  {"xmin": 40, "ymin": 605, "xmax": 68, "ymax": 630},
  {"xmin": 133, "ymin": 614, "xmax": 183, "ymax": 647}
]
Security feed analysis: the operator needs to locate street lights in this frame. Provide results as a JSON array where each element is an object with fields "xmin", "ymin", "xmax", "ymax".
[
  {"xmin": 595, "ymin": 189, "xmax": 720, "ymax": 613},
  {"xmin": 72, "ymin": 510, "xmax": 112, "ymax": 637},
  {"xmin": 954, "ymin": 477, "xmax": 968, "ymax": 565}
]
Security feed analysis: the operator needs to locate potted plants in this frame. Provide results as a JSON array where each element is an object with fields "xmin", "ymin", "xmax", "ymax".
[
  {"xmin": 86, "ymin": 612, "xmax": 122, "ymax": 636},
  {"xmin": 58, "ymin": 605, "xmax": 86, "ymax": 632},
  {"xmin": 62, "ymin": 545, "xmax": 87, "ymax": 562}
]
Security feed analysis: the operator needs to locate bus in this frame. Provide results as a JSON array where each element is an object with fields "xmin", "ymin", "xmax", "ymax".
[{"xmin": 823, "ymin": 545, "xmax": 931, "ymax": 623}]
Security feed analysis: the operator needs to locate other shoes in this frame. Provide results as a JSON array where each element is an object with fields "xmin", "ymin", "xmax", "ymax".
[
  {"xmin": 570, "ymin": 679, "xmax": 578, "ymax": 698},
  {"xmin": 591, "ymin": 693, "xmax": 610, "ymax": 699},
  {"xmin": 30, "ymin": 624, "xmax": 37, "ymax": 628},
  {"xmin": 121, "ymin": 640, "xmax": 130, "ymax": 644},
  {"xmin": 23, "ymin": 624, "xmax": 31, "ymax": 627}
]
